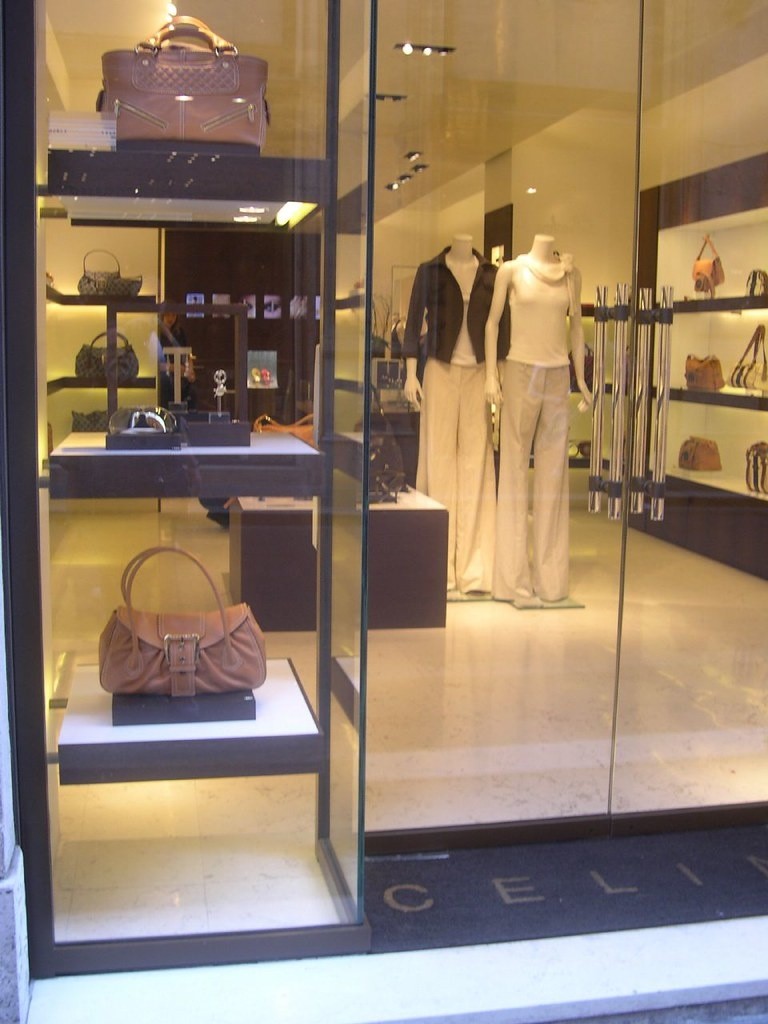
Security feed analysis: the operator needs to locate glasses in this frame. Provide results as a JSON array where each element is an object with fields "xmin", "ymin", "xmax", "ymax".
[{"xmin": 107, "ymin": 407, "xmax": 179, "ymax": 434}]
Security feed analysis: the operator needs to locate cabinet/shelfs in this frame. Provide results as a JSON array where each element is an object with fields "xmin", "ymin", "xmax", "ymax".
[
  {"xmin": 47, "ymin": 150, "xmax": 324, "ymax": 786},
  {"xmin": 333, "ymin": 181, "xmax": 364, "ymax": 733},
  {"xmin": 628, "ymin": 152, "xmax": 768, "ymax": 582},
  {"xmin": 39, "ymin": 209, "xmax": 157, "ymax": 395}
]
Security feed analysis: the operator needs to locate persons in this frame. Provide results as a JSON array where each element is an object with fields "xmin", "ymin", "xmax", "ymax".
[
  {"xmin": 151, "ymin": 301, "xmax": 195, "ymax": 400},
  {"xmin": 391, "ymin": 315, "xmax": 404, "ymax": 376},
  {"xmin": 401, "ymin": 232, "xmax": 510, "ymax": 596},
  {"xmin": 484, "ymin": 235, "xmax": 590, "ymax": 604}
]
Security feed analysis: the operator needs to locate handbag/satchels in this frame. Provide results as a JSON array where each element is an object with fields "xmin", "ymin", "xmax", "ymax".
[
  {"xmin": 102, "ymin": 17, "xmax": 276, "ymax": 153},
  {"xmin": 726, "ymin": 325, "xmax": 767, "ymax": 393},
  {"xmin": 692, "ymin": 235, "xmax": 724, "ymax": 299},
  {"xmin": 253, "ymin": 414, "xmax": 315, "ymax": 447},
  {"xmin": 77, "ymin": 251, "xmax": 144, "ymax": 296},
  {"xmin": 570, "ymin": 440, "xmax": 593, "ymax": 460},
  {"xmin": 678, "ymin": 436, "xmax": 722, "ymax": 471},
  {"xmin": 74, "ymin": 331, "xmax": 140, "ymax": 380},
  {"xmin": 368, "ymin": 382, "xmax": 404, "ymax": 493},
  {"xmin": 745, "ymin": 442, "xmax": 768, "ymax": 493},
  {"xmin": 744, "ymin": 269, "xmax": 768, "ymax": 296},
  {"xmin": 568, "ymin": 343, "xmax": 596, "ymax": 391},
  {"xmin": 69, "ymin": 410, "xmax": 108, "ymax": 433},
  {"xmin": 684, "ymin": 354, "xmax": 725, "ymax": 391},
  {"xmin": 372, "ymin": 311, "xmax": 385, "ymax": 358},
  {"xmin": 97, "ymin": 546, "xmax": 268, "ymax": 695}
]
